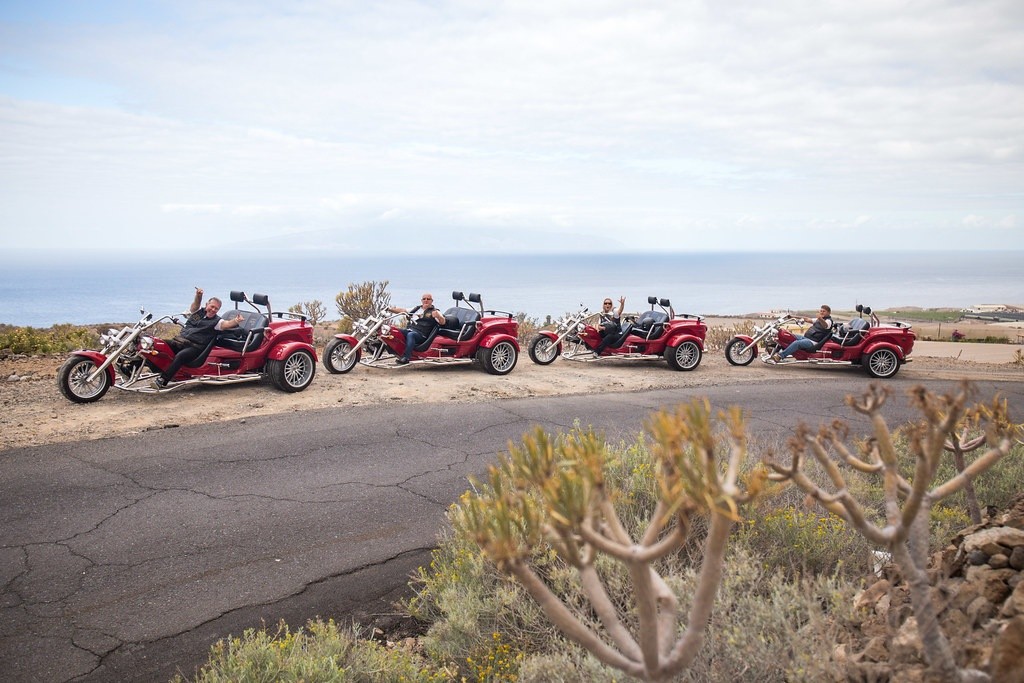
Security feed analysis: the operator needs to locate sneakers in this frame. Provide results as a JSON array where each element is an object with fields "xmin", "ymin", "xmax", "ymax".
[{"xmin": 395, "ymin": 357, "xmax": 410, "ymax": 365}]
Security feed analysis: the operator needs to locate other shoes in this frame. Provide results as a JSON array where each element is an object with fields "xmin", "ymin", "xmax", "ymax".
[
  {"xmin": 593, "ymin": 352, "xmax": 598, "ymax": 359},
  {"xmin": 150, "ymin": 378, "xmax": 163, "ymax": 391},
  {"xmin": 761, "ymin": 355, "xmax": 777, "ymax": 365},
  {"xmin": 121, "ymin": 364, "xmax": 135, "ymax": 378}
]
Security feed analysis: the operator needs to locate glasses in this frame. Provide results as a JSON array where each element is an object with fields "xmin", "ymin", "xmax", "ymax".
[
  {"xmin": 604, "ymin": 302, "xmax": 612, "ymax": 305},
  {"xmin": 422, "ymin": 297, "xmax": 432, "ymax": 300}
]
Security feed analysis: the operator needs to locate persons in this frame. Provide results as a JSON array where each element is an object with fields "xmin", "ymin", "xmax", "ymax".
[
  {"xmin": 114, "ymin": 287, "xmax": 244, "ymax": 390},
  {"xmin": 767, "ymin": 305, "xmax": 833, "ymax": 363},
  {"xmin": 592, "ymin": 296, "xmax": 626, "ymax": 358},
  {"xmin": 367, "ymin": 294, "xmax": 445, "ymax": 365}
]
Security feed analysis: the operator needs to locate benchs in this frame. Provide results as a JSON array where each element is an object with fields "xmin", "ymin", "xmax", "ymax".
[
  {"xmin": 631, "ymin": 296, "xmax": 670, "ymax": 341},
  {"xmin": 439, "ymin": 291, "xmax": 484, "ymax": 341},
  {"xmin": 215, "ymin": 291, "xmax": 272, "ymax": 352},
  {"xmin": 831, "ymin": 304, "xmax": 871, "ymax": 346}
]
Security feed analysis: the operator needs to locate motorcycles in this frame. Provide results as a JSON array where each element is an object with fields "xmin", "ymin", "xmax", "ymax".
[
  {"xmin": 55, "ymin": 290, "xmax": 319, "ymax": 403},
  {"xmin": 724, "ymin": 305, "xmax": 917, "ymax": 380},
  {"xmin": 322, "ymin": 290, "xmax": 520, "ymax": 376},
  {"xmin": 528, "ymin": 298, "xmax": 709, "ymax": 373}
]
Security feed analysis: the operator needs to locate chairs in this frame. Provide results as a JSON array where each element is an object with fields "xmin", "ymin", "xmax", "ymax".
[
  {"xmin": 186, "ymin": 330, "xmax": 217, "ymax": 367},
  {"xmin": 413, "ymin": 314, "xmax": 445, "ymax": 352},
  {"xmin": 803, "ymin": 330, "xmax": 833, "ymax": 353},
  {"xmin": 607, "ymin": 322, "xmax": 633, "ymax": 347}
]
